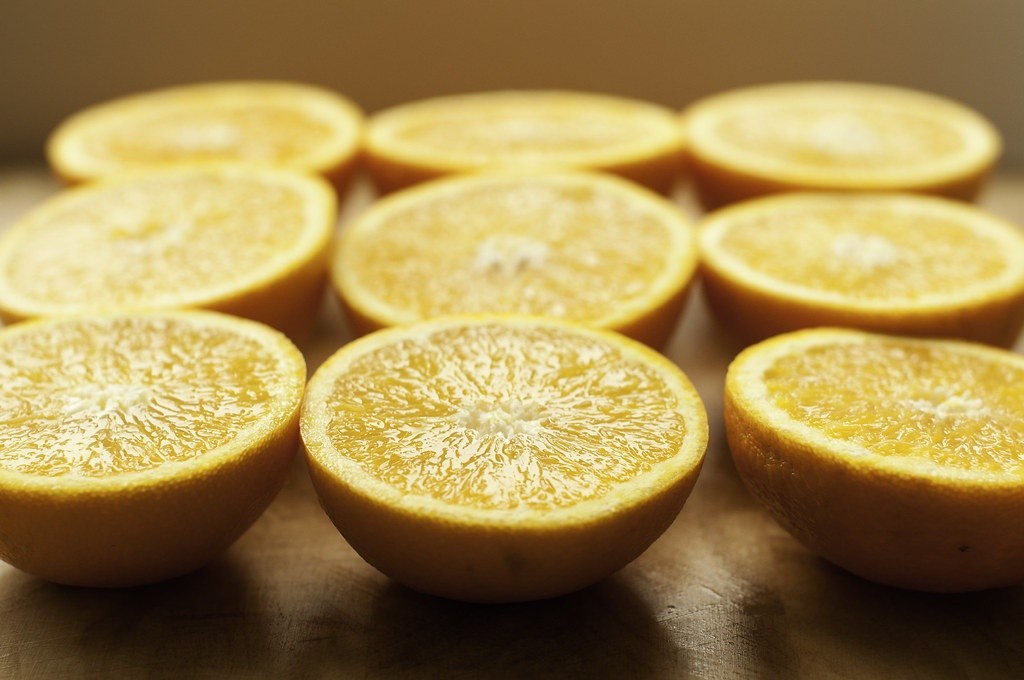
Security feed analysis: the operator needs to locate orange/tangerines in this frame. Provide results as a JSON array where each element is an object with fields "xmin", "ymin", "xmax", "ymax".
[{"xmin": 0, "ymin": 77, "xmax": 1023, "ymax": 605}]
